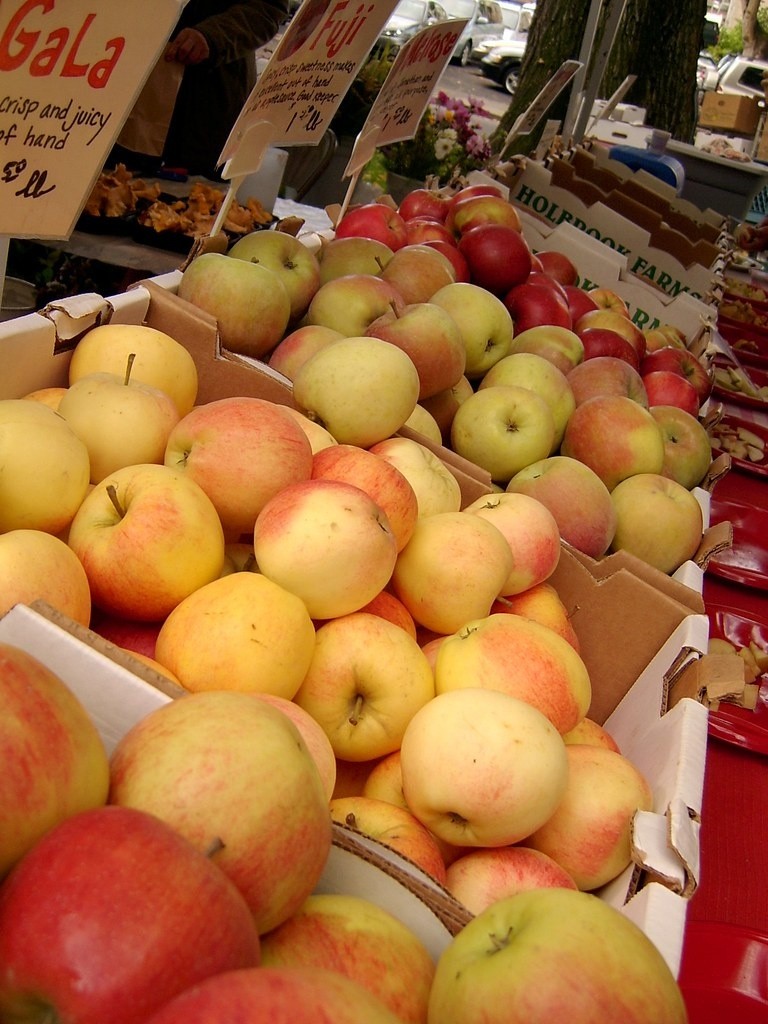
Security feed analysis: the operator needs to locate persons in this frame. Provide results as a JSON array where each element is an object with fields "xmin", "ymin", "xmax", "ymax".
[
  {"xmin": 735, "ymin": 214, "xmax": 768, "ymax": 252},
  {"xmin": 8, "ymin": 0, "xmax": 292, "ymax": 308}
]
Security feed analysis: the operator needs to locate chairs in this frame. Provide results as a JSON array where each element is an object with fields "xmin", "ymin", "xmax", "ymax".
[{"xmin": 279, "ymin": 129, "xmax": 339, "ymax": 202}]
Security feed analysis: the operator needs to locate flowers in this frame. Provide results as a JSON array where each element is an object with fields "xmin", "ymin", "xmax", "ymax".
[{"xmin": 422, "ymin": 82, "xmax": 515, "ymax": 169}]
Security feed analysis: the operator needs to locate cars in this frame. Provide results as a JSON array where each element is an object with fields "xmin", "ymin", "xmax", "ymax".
[
  {"xmin": 370, "ymin": 1, "xmax": 532, "ymax": 95},
  {"xmin": 693, "ymin": 51, "xmax": 768, "ymax": 123}
]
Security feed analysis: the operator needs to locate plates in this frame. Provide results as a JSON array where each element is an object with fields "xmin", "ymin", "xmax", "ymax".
[{"xmin": 731, "ymin": 252, "xmax": 765, "ymax": 271}]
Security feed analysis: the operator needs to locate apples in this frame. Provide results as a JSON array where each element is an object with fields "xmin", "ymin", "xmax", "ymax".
[
  {"xmin": 177, "ymin": 182, "xmax": 714, "ymax": 573},
  {"xmin": 0, "ymin": 322, "xmax": 691, "ymax": 1024}
]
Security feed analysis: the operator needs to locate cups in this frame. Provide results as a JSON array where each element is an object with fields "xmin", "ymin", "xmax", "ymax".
[
  {"xmin": 650, "ymin": 128, "xmax": 672, "ymax": 156},
  {"xmin": 234, "ymin": 148, "xmax": 288, "ymax": 213}
]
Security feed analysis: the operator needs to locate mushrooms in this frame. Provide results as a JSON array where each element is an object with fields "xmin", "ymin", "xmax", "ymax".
[{"xmin": 82, "ymin": 163, "xmax": 271, "ymax": 236}]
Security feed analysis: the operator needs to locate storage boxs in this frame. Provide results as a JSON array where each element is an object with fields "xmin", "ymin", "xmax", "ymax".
[{"xmin": 0, "ymin": 92, "xmax": 760, "ymax": 1024}]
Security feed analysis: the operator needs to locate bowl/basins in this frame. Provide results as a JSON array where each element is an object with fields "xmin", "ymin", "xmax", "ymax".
[{"xmin": 75, "ymin": 193, "xmax": 279, "ymax": 255}]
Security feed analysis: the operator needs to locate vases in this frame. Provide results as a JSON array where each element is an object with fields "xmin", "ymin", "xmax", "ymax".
[{"xmin": 386, "ymin": 169, "xmax": 428, "ymax": 208}]
